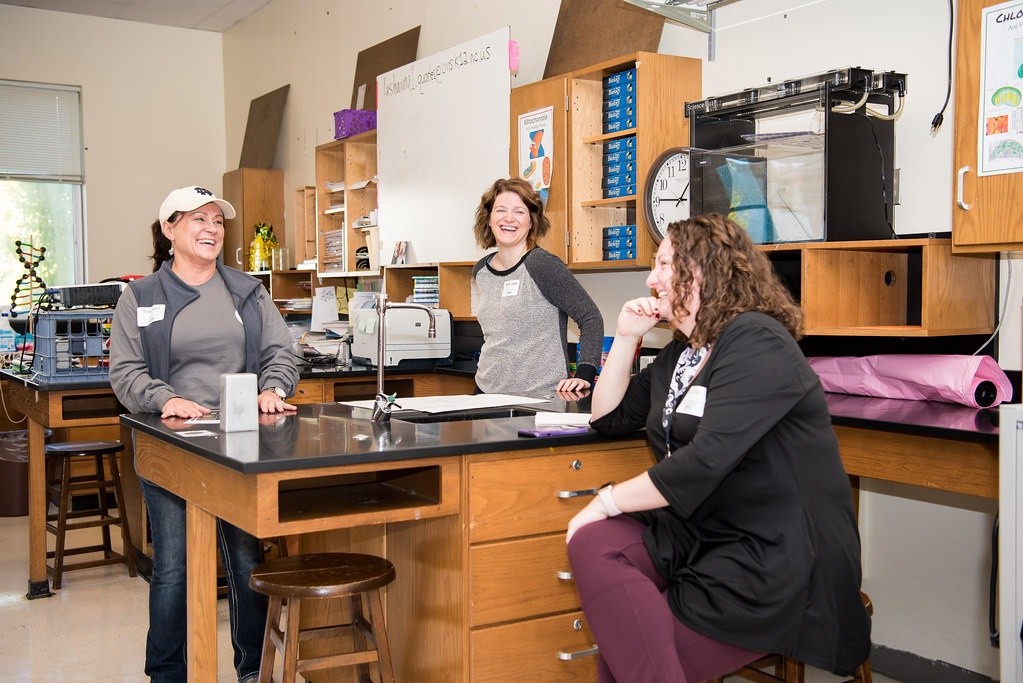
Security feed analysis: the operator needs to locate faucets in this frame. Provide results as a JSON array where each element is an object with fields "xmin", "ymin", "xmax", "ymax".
[{"xmin": 372, "ymin": 293, "xmax": 437, "ymax": 423}]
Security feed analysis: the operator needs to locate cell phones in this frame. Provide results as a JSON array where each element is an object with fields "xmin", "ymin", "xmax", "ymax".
[{"xmin": 518, "ymin": 425, "xmax": 589, "ymax": 438}]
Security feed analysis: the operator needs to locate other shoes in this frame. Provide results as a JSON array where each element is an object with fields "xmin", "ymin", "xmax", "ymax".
[{"xmin": 238, "ymin": 673, "xmax": 275, "ymax": 683}]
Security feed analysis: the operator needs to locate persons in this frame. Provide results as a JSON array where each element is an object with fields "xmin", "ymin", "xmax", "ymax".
[
  {"xmin": 161, "ymin": 411, "xmax": 299, "ymax": 461},
  {"xmin": 109, "ymin": 185, "xmax": 304, "ymax": 683},
  {"xmin": 564, "ymin": 212, "xmax": 868, "ymax": 683},
  {"xmin": 470, "ymin": 178, "xmax": 604, "ymax": 398}
]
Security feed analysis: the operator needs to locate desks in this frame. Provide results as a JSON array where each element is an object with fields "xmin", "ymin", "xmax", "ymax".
[{"xmin": 0, "ymin": 352, "xmax": 1000, "ymax": 683}]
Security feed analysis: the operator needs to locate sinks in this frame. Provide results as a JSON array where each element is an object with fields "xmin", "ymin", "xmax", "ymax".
[{"xmin": 391, "ymin": 406, "xmax": 537, "ymax": 423}]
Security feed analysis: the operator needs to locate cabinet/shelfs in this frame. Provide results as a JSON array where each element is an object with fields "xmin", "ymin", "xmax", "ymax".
[{"xmin": 223, "ymin": 0, "xmax": 1023, "ymax": 340}]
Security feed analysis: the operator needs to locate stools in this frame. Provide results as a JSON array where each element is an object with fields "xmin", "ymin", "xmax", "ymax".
[
  {"xmin": 44, "ymin": 439, "xmax": 138, "ymax": 589},
  {"xmin": 712, "ymin": 590, "xmax": 875, "ymax": 683},
  {"xmin": 248, "ymin": 552, "xmax": 397, "ymax": 683}
]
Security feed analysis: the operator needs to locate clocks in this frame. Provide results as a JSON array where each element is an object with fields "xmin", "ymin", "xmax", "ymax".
[{"xmin": 643, "ymin": 146, "xmax": 689, "ymax": 247}]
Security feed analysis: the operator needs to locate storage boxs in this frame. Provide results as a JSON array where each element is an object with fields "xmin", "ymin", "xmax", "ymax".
[
  {"xmin": 690, "ymin": 80, "xmax": 894, "ymax": 246},
  {"xmin": 31, "ymin": 310, "xmax": 115, "ymax": 387},
  {"xmin": 333, "ymin": 109, "xmax": 377, "ymax": 139}
]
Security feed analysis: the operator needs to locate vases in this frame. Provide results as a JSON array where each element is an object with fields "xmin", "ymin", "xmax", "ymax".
[{"xmin": 249, "ymin": 232, "xmax": 265, "ymax": 272}]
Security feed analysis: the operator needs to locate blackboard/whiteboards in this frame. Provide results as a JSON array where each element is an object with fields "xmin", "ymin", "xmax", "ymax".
[{"xmin": 376, "ymin": 24, "xmax": 512, "ymax": 266}]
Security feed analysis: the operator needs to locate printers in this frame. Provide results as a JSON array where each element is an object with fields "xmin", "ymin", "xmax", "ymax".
[{"xmin": 350, "ymin": 302, "xmax": 453, "ymax": 370}]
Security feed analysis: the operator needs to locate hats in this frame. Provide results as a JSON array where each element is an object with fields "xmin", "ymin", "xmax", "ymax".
[{"xmin": 158, "ymin": 186, "xmax": 236, "ymax": 233}]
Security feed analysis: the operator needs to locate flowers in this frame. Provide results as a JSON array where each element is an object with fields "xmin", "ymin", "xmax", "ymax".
[{"xmin": 254, "ymin": 220, "xmax": 278, "ymax": 257}]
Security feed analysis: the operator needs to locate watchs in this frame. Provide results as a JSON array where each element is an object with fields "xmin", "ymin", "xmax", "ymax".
[
  {"xmin": 274, "ymin": 416, "xmax": 286, "ymax": 427},
  {"xmin": 264, "ymin": 387, "xmax": 287, "ymax": 402},
  {"xmin": 597, "ymin": 485, "xmax": 622, "ymax": 517}
]
